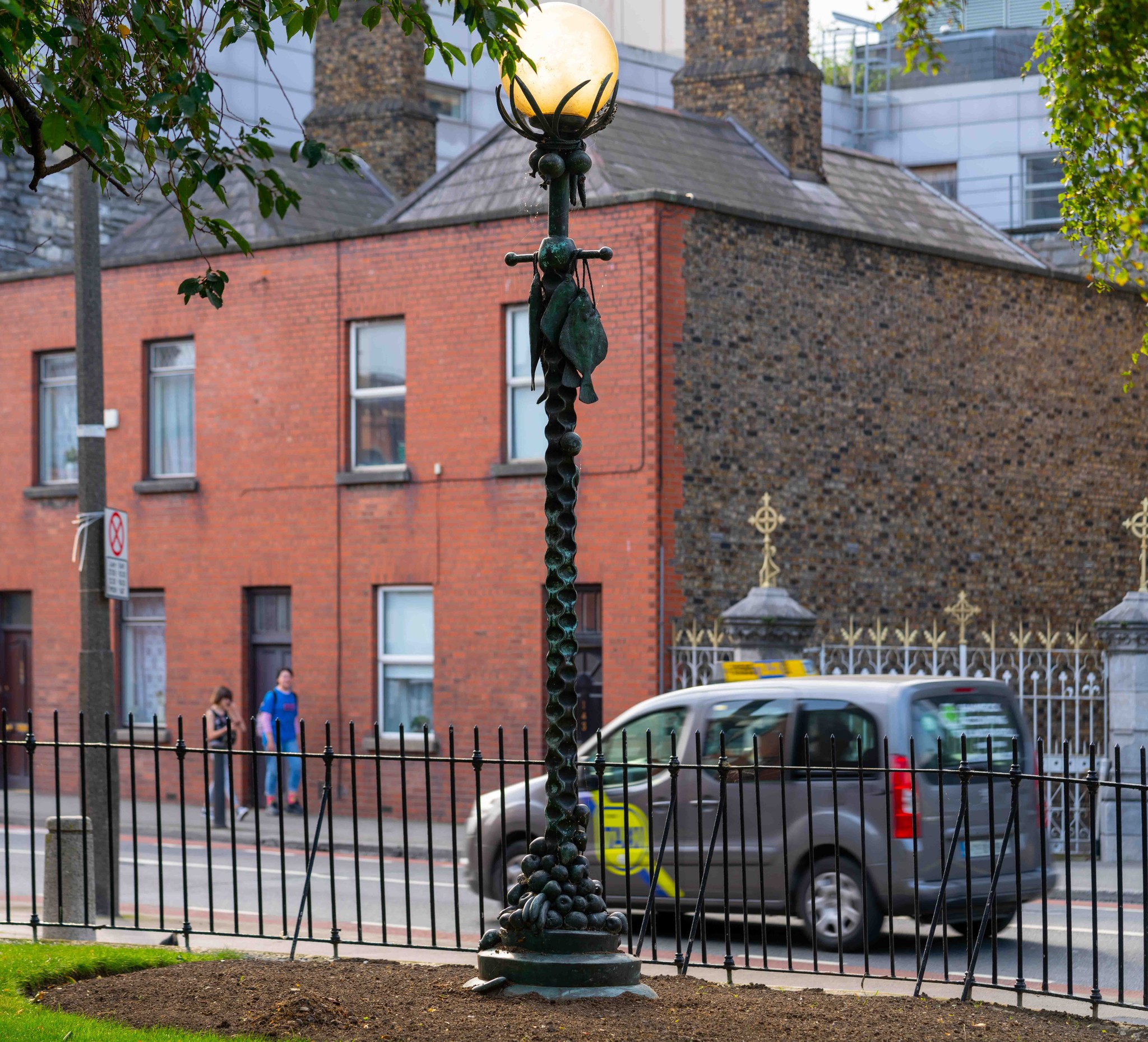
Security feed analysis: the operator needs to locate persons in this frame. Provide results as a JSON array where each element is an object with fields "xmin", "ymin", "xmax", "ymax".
[
  {"xmin": 734, "ymin": 719, "xmax": 787, "ymax": 781},
  {"xmin": 258, "ymin": 668, "xmax": 304, "ymax": 817},
  {"xmin": 201, "ymin": 685, "xmax": 250, "ymax": 821}
]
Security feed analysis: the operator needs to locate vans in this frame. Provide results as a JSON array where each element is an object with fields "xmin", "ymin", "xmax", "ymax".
[{"xmin": 460, "ymin": 673, "xmax": 1060, "ymax": 954}]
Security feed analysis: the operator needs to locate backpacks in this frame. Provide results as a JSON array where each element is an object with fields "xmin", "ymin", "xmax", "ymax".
[{"xmin": 256, "ymin": 691, "xmax": 278, "ymax": 736}]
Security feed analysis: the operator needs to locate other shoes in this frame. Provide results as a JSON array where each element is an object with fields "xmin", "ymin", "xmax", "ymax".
[
  {"xmin": 286, "ymin": 802, "xmax": 303, "ymax": 815},
  {"xmin": 201, "ymin": 806, "xmax": 215, "ymax": 815},
  {"xmin": 237, "ymin": 808, "xmax": 247, "ymax": 821},
  {"xmin": 265, "ymin": 803, "xmax": 280, "ymax": 818}
]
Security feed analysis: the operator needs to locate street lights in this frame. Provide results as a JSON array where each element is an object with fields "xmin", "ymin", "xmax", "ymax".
[{"xmin": 468, "ymin": 2, "xmax": 656, "ymax": 991}]
